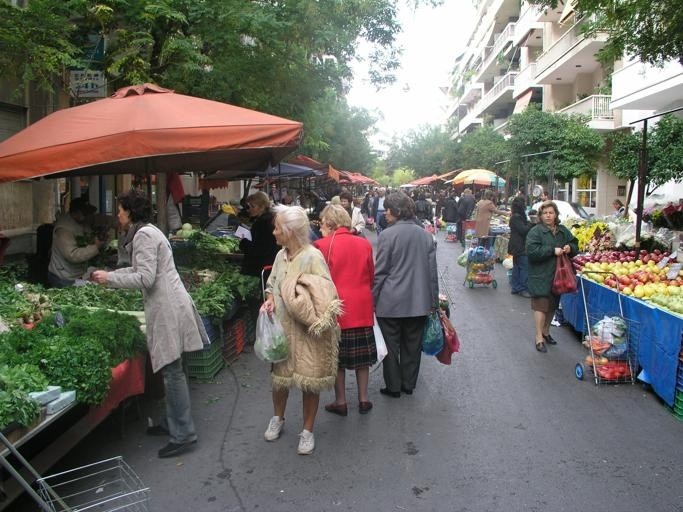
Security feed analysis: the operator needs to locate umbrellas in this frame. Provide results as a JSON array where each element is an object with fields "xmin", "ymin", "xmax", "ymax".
[{"xmin": 0, "ymin": 81, "xmax": 304, "ymax": 229}]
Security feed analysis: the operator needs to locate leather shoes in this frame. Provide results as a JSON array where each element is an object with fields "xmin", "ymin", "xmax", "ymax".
[
  {"xmin": 359, "ymin": 401, "xmax": 373, "ymax": 414},
  {"xmin": 542, "ymin": 332, "xmax": 556, "ymax": 344},
  {"xmin": 519, "ymin": 289, "xmax": 531, "ymax": 298},
  {"xmin": 536, "ymin": 341, "xmax": 547, "ymax": 352},
  {"xmin": 401, "ymin": 385, "xmax": 413, "ymax": 395},
  {"xmin": 380, "ymin": 387, "xmax": 400, "ymax": 398},
  {"xmin": 325, "ymin": 402, "xmax": 347, "ymax": 416},
  {"xmin": 147, "ymin": 425, "xmax": 168, "ymax": 436},
  {"xmin": 159, "ymin": 439, "xmax": 198, "ymax": 458}
]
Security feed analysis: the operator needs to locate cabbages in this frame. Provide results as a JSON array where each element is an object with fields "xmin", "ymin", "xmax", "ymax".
[{"xmin": 176, "ymin": 222, "xmax": 196, "ymax": 237}]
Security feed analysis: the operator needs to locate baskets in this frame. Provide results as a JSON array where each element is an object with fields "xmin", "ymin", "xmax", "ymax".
[
  {"xmin": 200, "ymin": 314, "xmax": 218, "ymax": 342},
  {"xmin": 676, "ymin": 361, "xmax": 683, "ymax": 391},
  {"xmin": 182, "ymin": 338, "xmax": 225, "ymax": 380},
  {"xmin": 664, "ymin": 390, "xmax": 683, "ymax": 421}
]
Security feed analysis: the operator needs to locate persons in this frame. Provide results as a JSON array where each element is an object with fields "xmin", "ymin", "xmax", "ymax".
[
  {"xmin": 237, "ymin": 189, "xmax": 281, "ymax": 335},
  {"xmin": 91, "ymin": 185, "xmax": 212, "ymax": 458},
  {"xmin": 306, "ymin": 204, "xmax": 379, "ymax": 418},
  {"xmin": 255, "ymin": 201, "xmax": 342, "ymax": 458},
  {"xmin": 366, "ymin": 188, "xmax": 441, "ymax": 398},
  {"xmin": 243, "ymin": 180, "xmax": 636, "ymax": 297},
  {"xmin": 524, "ymin": 199, "xmax": 580, "ymax": 353},
  {"xmin": 42, "ymin": 195, "xmax": 104, "ymax": 290}
]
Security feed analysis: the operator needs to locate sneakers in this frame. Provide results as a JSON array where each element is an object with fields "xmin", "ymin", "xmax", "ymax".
[
  {"xmin": 297, "ymin": 428, "xmax": 315, "ymax": 456},
  {"xmin": 264, "ymin": 415, "xmax": 286, "ymax": 442}
]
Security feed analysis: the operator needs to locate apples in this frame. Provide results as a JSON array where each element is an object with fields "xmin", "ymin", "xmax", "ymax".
[
  {"xmin": 581, "ymin": 260, "xmax": 683, "ymax": 284},
  {"xmin": 571, "ymin": 249, "xmax": 679, "ymax": 273},
  {"xmin": 650, "ymin": 294, "xmax": 683, "ymax": 315},
  {"xmin": 604, "ymin": 272, "xmax": 683, "ymax": 290}
]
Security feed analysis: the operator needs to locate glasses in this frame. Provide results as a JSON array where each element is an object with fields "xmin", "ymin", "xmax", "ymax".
[{"xmin": 318, "ymin": 220, "xmax": 327, "ymax": 228}]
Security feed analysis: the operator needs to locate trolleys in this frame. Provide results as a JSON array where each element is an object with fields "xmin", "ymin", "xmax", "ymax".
[
  {"xmin": 444, "ymin": 221, "xmax": 457, "ymax": 243},
  {"xmin": 574, "ymin": 271, "xmax": 636, "ymax": 388},
  {"xmin": 0, "ymin": 431, "xmax": 150, "ymax": 511},
  {"xmin": 461, "ymin": 235, "xmax": 498, "ymax": 288}
]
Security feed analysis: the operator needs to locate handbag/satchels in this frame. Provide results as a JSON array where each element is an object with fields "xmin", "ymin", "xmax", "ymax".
[{"xmin": 368, "ymin": 310, "xmax": 389, "ymax": 375}]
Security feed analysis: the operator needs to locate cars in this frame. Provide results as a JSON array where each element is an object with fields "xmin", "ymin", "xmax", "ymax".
[{"xmin": 533, "ymin": 200, "xmax": 593, "ymax": 221}]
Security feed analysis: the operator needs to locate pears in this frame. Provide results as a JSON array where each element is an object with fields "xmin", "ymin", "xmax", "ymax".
[{"xmin": 623, "ymin": 282, "xmax": 683, "ymax": 298}]
